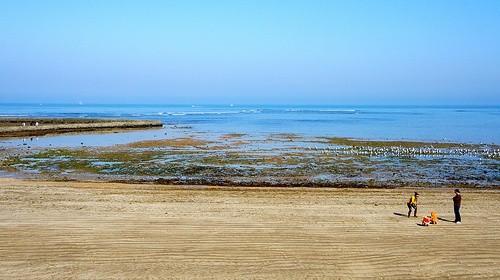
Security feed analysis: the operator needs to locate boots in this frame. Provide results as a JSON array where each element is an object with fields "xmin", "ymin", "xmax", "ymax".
[
  {"xmin": 408, "ymin": 212, "xmax": 411, "ymax": 218},
  {"xmin": 414, "ymin": 211, "xmax": 417, "ymax": 217}
]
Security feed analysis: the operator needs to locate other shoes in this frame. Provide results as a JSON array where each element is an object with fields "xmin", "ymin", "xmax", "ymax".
[{"xmin": 456, "ymin": 221, "xmax": 461, "ymax": 224}]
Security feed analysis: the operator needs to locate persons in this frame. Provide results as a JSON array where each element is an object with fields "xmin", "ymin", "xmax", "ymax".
[
  {"xmin": 421, "ymin": 215, "xmax": 430, "ymax": 226},
  {"xmin": 407, "ymin": 192, "xmax": 419, "ymax": 218},
  {"xmin": 430, "ymin": 211, "xmax": 438, "ymax": 224},
  {"xmin": 453, "ymin": 189, "xmax": 462, "ymax": 224}
]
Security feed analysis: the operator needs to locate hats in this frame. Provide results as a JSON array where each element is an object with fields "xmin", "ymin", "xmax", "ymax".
[
  {"xmin": 414, "ymin": 192, "xmax": 418, "ymax": 198},
  {"xmin": 455, "ymin": 189, "xmax": 461, "ymax": 193}
]
